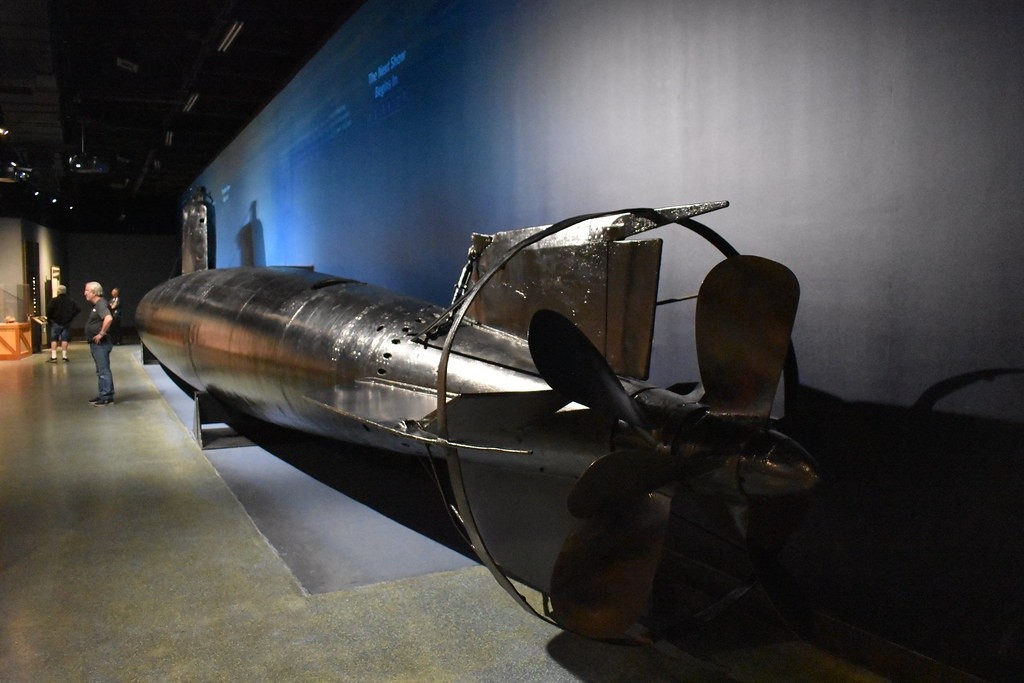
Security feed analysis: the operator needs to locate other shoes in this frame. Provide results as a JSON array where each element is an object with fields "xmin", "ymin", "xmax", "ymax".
[
  {"xmin": 46, "ymin": 357, "xmax": 58, "ymax": 363},
  {"xmin": 63, "ymin": 358, "xmax": 69, "ymax": 362},
  {"xmin": 89, "ymin": 397, "xmax": 100, "ymax": 403},
  {"xmin": 95, "ymin": 400, "xmax": 114, "ymax": 406}
]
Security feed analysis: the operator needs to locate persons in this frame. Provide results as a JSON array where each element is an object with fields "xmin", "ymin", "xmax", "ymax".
[
  {"xmin": 107, "ymin": 287, "xmax": 120, "ymax": 346},
  {"xmin": 46, "ymin": 285, "xmax": 82, "ymax": 362},
  {"xmin": 84, "ymin": 281, "xmax": 115, "ymax": 407}
]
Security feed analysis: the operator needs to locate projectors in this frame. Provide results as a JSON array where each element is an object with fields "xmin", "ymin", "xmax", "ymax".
[{"xmin": 69, "ymin": 158, "xmax": 110, "ymax": 175}]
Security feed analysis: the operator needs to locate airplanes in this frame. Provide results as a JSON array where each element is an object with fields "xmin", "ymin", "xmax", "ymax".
[{"xmin": 0, "ymin": 152, "xmax": 111, "ymax": 192}]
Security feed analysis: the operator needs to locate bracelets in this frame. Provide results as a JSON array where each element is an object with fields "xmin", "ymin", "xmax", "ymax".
[{"xmin": 100, "ymin": 332, "xmax": 103, "ymax": 336}]
